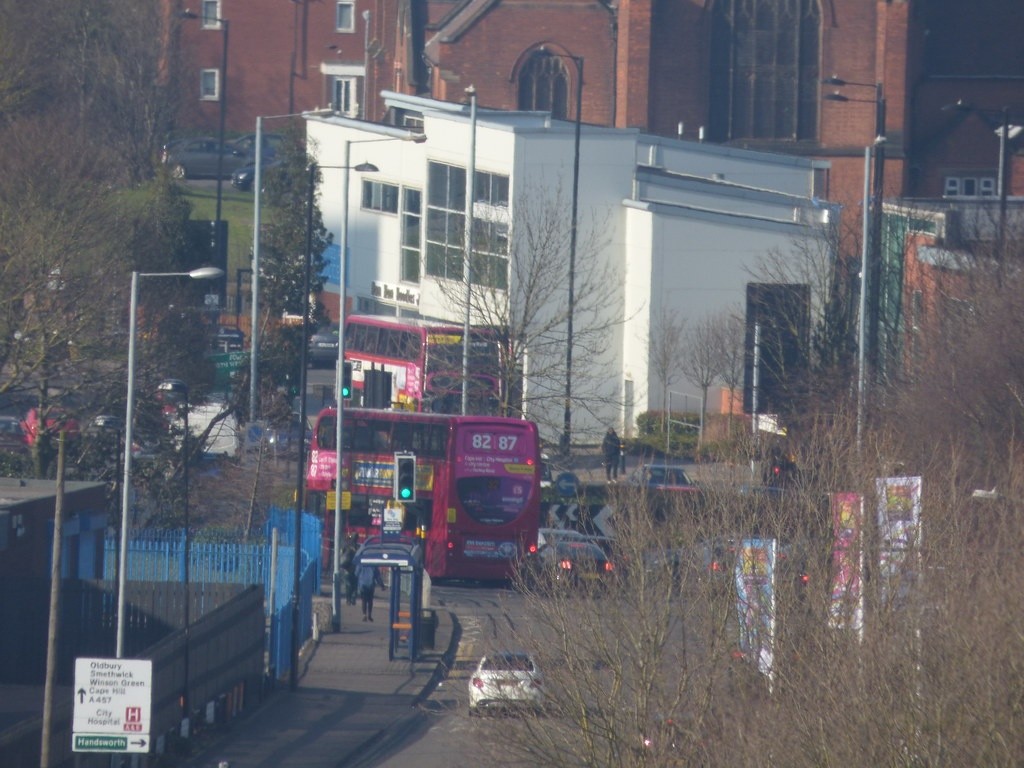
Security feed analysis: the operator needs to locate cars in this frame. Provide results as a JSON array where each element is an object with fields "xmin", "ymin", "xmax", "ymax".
[
  {"xmin": 158, "ymin": 131, "xmax": 306, "ymax": 192},
  {"xmin": 351, "ymin": 533, "xmax": 427, "ymax": 599},
  {"xmin": 209, "ymin": 304, "xmax": 341, "ymax": 364},
  {"xmin": 464, "ymin": 652, "xmax": 557, "ymax": 717},
  {"xmin": 0, "ymin": 380, "xmax": 245, "ymax": 463},
  {"xmin": 541, "ymin": 466, "xmax": 834, "ymax": 604}
]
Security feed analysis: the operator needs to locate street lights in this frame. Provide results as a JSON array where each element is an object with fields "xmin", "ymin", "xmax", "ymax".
[
  {"xmin": 859, "ymin": 134, "xmax": 891, "ymax": 458},
  {"xmin": 114, "ymin": 266, "xmax": 226, "ymax": 659},
  {"xmin": 250, "ymin": 108, "xmax": 334, "ymax": 419},
  {"xmin": 817, "ymin": 74, "xmax": 906, "ymax": 368},
  {"xmin": 287, "ymin": 162, "xmax": 379, "ymax": 692},
  {"xmin": 180, "ymin": 9, "xmax": 231, "ymax": 265},
  {"xmin": 331, "ymin": 135, "xmax": 429, "ymax": 634},
  {"xmin": 532, "ymin": 44, "xmax": 586, "ymax": 461},
  {"xmin": 460, "ymin": 85, "xmax": 477, "ymax": 411}
]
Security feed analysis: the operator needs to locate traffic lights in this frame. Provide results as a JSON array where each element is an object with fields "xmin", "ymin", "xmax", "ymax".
[
  {"xmin": 342, "ymin": 362, "xmax": 353, "ymax": 401},
  {"xmin": 392, "ymin": 453, "xmax": 418, "ymax": 503}
]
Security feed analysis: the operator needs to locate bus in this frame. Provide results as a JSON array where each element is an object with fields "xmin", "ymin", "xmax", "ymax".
[
  {"xmin": 306, "ymin": 407, "xmax": 543, "ymax": 585},
  {"xmin": 338, "ymin": 316, "xmax": 510, "ymax": 413}
]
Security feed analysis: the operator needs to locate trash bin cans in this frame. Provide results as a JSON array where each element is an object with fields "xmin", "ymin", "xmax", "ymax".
[{"xmin": 419, "ymin": 608, "xmax": 436, "ymax": 650}]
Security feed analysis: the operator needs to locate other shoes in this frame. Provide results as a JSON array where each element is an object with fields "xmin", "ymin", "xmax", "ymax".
[
  {"xmin": 368, "ymin": 616, "xmax": 373, "ymax": 622},
  {"xmin": 612, "ymin": 479, "xmax": 617, "ymax": 483},
  {"xmin": 608, "ymin": 480, "xmax": 611, "ymax": 484},
  {"xmin": 363, "ymin": 617, "xmax": 367, "ymax": 622}
]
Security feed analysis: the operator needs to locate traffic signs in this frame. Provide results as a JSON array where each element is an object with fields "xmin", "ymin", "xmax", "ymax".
[{"xmin": 69, "ymin": 660, "xmax": 153, "ymax": 756}]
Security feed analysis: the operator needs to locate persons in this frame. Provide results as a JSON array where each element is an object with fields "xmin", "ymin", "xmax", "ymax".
[
  {"xmin": 354, "ymin": 562, "xmax": 385, "ymax": 623},
  {"xmin": 602, "ymin": 426, "xmax": 621, "ymax": 484},
  {"xmin": 335, "ymin": 531, "xmax": 362, "ymax": 606}
]
